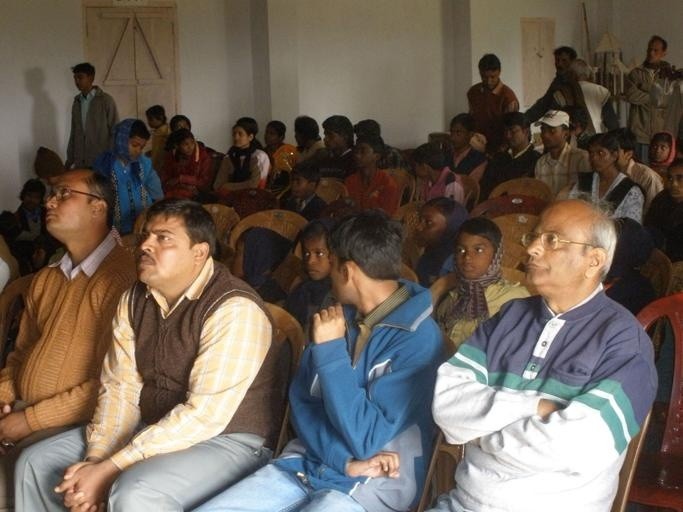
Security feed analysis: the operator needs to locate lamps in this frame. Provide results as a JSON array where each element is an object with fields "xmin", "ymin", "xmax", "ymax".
[{"xmin": 594, "ymin": 32, "xmax": 615, "ymax": 86}]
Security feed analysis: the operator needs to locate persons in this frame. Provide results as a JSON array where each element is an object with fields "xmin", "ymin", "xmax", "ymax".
[
  {"xmin": 13, "ymin": 196, "xmax": 286, "ymax": 512},
  {"xmin": 0, "ymin": 104, "xmax": 682, "ymax": 351},
  {"xmin": 186, "ymin": 208, "xmax": 445, "ymax": 512},
  {"xmin": 423, "ymin": 198, "xmax": 659, "ymax": 512},
  {"xmin": 64, "ymin": 63, "xmax": 119, "ymax": 169},
  {"xmin": 468, "ymin": 35, "xmax": 683, "ymax": 159},
  {"xmin": 0, "ymin": 166, "xmax": 140, "ymax": 512}
]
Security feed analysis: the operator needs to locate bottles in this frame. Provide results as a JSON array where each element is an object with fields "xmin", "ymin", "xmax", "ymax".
[{"xmin": 591, "ymin": 66, "xmax": 599, "ymax": 82}]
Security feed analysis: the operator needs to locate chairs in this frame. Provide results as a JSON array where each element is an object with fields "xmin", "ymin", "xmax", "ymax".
[
  {"xmin": 264, "ymin": 301, "xmax": 305, "ymax": 448},
  {"xmin": 634, "ymin": 292, "xmax": 683, "ymax": 512},
  {"xmin": 419, "ymin": 407, "xmax": 652, "ymax": 512},
  {"xmin": 0, "ymin": 275, "xmax": 32, "ymax": 361},
  {"xmin": 274, "ymin": 301, "xmax": 459, "ymax": 511}
]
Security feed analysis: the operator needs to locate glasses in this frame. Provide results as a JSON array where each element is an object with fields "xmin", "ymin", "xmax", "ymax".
[
  {"xmin": 44, "ymin": 188, "xmax": 102, "ymax": 203},
  {"xmin": 522, "ymin": 232, "xmax": 604, "ymax": 250}
]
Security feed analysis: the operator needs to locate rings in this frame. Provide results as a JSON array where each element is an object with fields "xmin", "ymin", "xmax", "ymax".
[{"xmin": 2, "ymin": 441, "xmax": 15, "ymax": 448}]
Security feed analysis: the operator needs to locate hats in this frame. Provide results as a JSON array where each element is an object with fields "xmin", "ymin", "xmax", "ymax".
[{"xmin": 535, "ymin": 110, "xmax": 569, "ymax": 128}]
